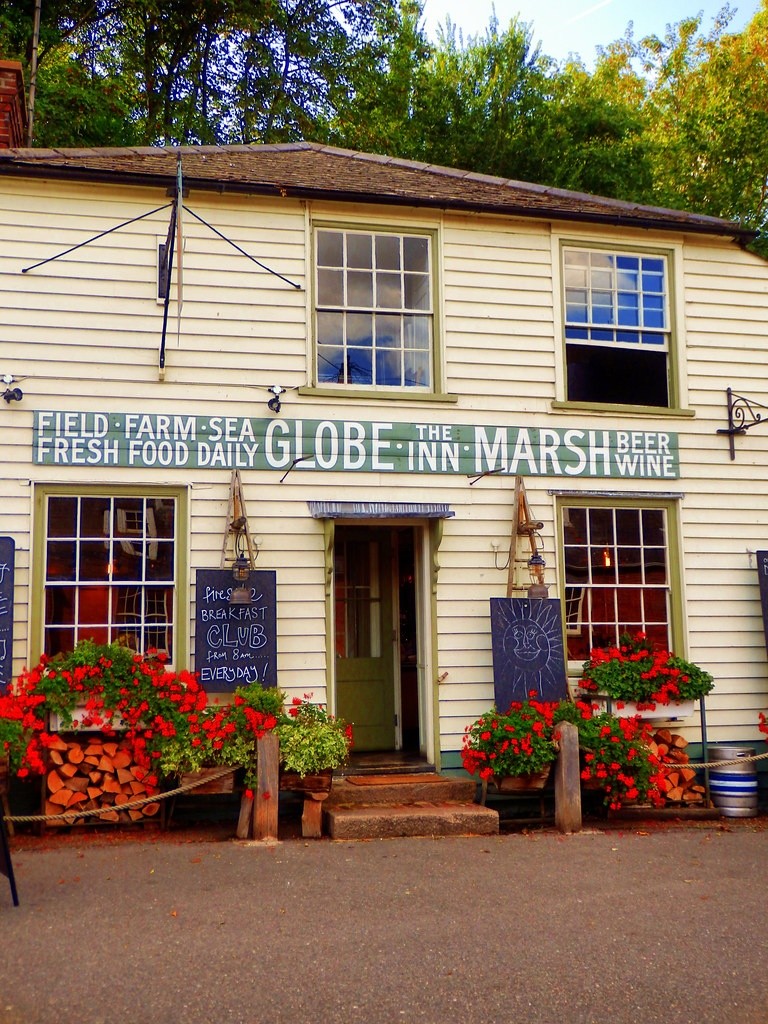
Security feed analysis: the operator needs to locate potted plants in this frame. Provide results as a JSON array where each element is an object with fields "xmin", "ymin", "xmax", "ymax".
[{"xmin": 278, "ymin": 723, "xmax": 349, "ymax": 791}]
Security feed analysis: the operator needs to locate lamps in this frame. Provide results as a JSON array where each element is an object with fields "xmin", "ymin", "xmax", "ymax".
[
  {"xmin": 527, "ymin": 530, "xmax": 549, "ymax": 599},
  {"xmin": 224, "ymin": 556, "xmax": 254, "ymax": 605},
  {"xmin": 260, "ymin": 387, "xmax": 287, "ymax": 416},
  {"xmin": 1, "ymin": 373, "xmax": 24, "ymax": 402}
]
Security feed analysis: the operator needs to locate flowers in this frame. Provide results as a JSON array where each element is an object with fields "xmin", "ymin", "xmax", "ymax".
[
  {"xmin": 0, "ymin": 639, "xmax": 273, "ymax": 800},
  {"xmin": 462, "ymin": 690, "xmax": 666, "ymax": 812},
  {"xmin": 577, "ymin": 632, "xmax": 687, "ymax": 711}
]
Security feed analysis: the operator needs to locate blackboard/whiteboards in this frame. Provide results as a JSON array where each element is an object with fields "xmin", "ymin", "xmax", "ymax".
[
  {"xmin": 0, "ymin": 535, "xmax": 17, "ymax": 698},
  {"xmin": 490, "ymin": 597, "xmax": 568, "ymax": 712},
  {"xmin": 195, "ymin": 568, "xmax": 278, "ymax": 695}
]
